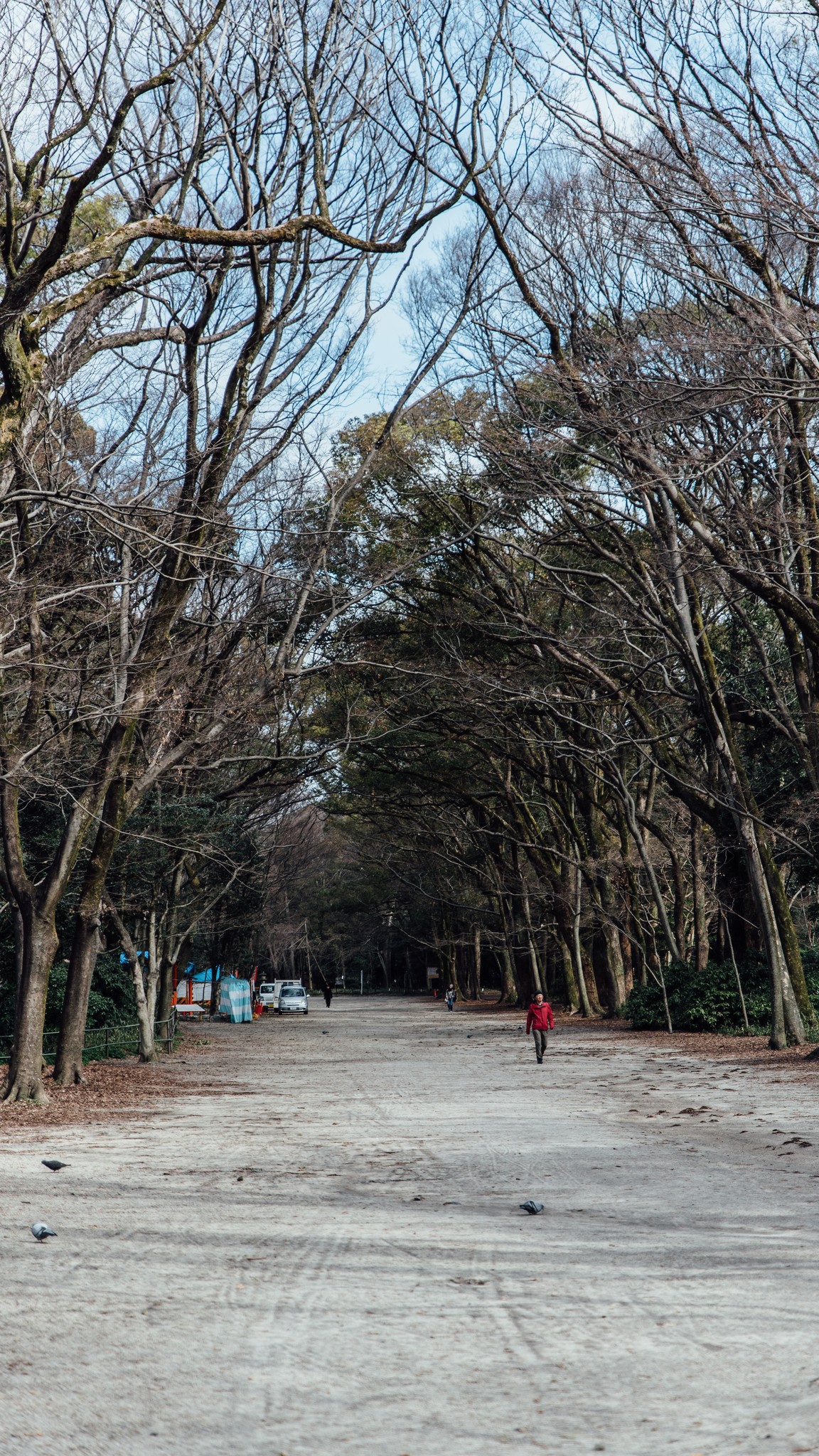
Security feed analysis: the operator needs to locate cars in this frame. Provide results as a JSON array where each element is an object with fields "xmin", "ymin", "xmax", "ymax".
[{"xmin": 275, "ymin": 986, "xmax": 310, "ymax": 1015}]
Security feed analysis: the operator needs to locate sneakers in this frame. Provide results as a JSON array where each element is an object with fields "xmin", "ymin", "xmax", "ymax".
[
  {"xmin": 448, "ymin": 1009, "xmax": 450, "ymax": 1011},
  {"xmin": 450, "ymin": 1011, "xmax": 453, "ymax": 1012}
]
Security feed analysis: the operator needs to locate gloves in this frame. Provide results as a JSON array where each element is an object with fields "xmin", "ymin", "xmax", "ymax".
[{"xmin": 331, "ymin": 997, "xmax": 333, "ymax": 999}]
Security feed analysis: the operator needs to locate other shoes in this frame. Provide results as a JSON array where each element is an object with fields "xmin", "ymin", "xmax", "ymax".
[
  {"xmin": 537, "ymin": 1059, "xmax": 543, "ymax": 1064},
  {"xmin": 328, "ymin": 1006, "xmax": 330, "ymax": 1008},
  {"xmin": 326, "ymin": 1005, "xmax": 328, "ymax": 1007}
]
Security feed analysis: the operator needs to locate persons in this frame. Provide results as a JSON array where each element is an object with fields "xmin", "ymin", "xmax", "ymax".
[
  {"xmin": 252, "ymin": 991, "xmax": 256, "ymax": 1007},
  {"xmin": 324, "ymin": 985, "xmax": 333, "ymax": 1008},
  {"xmin": 526, "ymin": 990, "xmax": 554, "ymax": 1063},
  {"xmin": 446, "ymin": 983, "xmax": 457, "ymax": 1011}
]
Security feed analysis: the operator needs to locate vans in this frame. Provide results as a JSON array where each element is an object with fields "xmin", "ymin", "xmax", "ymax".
[{"xmin": 260, "ymin": 983, "xmax": 275, "ymax": 1012}]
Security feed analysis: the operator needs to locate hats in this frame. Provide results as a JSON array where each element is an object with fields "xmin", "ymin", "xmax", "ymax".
[
  {"xmin": 534, "ymin": 990, "xmax": 543, "ymax": 997},
  {"xmin": 449, "ymin": 984, "xmax": 453, "ymax": 988}
]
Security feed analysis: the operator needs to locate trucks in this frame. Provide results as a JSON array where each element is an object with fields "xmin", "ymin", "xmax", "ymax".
[{"xmin": 274, "ymin": 978, "xmax": 302, "ymax": 1013}]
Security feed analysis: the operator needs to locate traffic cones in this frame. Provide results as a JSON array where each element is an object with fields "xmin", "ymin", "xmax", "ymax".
[{"xmin": 255, "ymin": 1001, "xmax": 263, "ymax": 1018}]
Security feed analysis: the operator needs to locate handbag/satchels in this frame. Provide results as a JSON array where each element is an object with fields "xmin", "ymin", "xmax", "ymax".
[{"xmin": 444, "ymin": 998, "xmax": 448, "ymax": 1002}]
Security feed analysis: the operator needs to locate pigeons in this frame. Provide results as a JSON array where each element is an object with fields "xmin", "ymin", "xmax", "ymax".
[
  {"xmin": 30, "ymin": 1223, "xmax": 57, "ymax": 1243},
  {"xmin": 519, "ymin": 1201, "xmax": 544, "ymax": 1216},
  {"xmin": 41, "ymin": 1160, "xmax": 72, "ymax": 1173}
]
